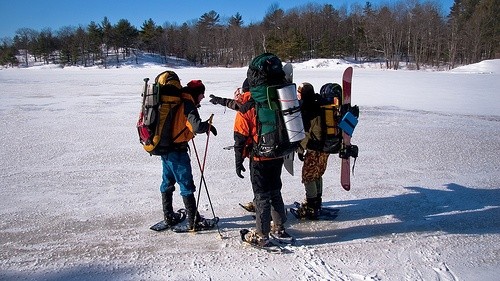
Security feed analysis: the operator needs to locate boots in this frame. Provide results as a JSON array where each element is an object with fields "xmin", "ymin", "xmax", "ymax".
[
  {"xmin": 290, "ymin": 198, "xmax": 322, "ymax": 220},
  {"xmin": 183, "ymin": 197, "xmax": 214, "ymax": 231},
  {"xmin": 161, "ymin": 191, "xmax": 183, "ymax": 226}
]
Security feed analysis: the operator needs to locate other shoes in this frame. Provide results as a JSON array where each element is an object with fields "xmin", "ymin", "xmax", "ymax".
[
  {"xmin": 246, "ymin": 202, "xmax": 257, "ymax": 211},
  {"xmin": 245, "ymin": 229, "xmax": 270, "ymax": 247},
  {"xmin": 271, "ymin": 224, "xmax": 287, "ymax": 238}
]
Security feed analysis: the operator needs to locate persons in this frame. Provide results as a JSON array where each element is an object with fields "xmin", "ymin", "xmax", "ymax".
[
  {"xmin": 160, "ymin": 80, "xmax": 218, "ymax": 229},
  {"xmin": 295, "ymin": 83, "xmax": 330, "ymax": 220},
  {"xmin": 209, "ymin": 78, "xmax": 287, "ymax": 245}
]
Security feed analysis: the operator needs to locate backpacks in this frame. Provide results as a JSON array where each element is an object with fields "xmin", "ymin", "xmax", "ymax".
[
  {"xmin": 137, "ymin": 71, "xmax": 187, "ymax": 156},
  {"xmin": 247, "ymin": 53, "xmax": 302, "ymax": 158},
  {"xmin": 310, "ymin": 83, "xmax": 342, "ymax": 153}
]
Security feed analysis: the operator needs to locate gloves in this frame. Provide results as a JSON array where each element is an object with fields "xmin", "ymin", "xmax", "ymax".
[
  {"xmin": 209, "ymin": 95, "xmax": 227, "ymax": 106},
  {"xmin": 203, "ymin": 122, "xmax": 217, "ymax": 136},
  {"xmin": 236, "ymin": 163, "xmax": 246, "ymax": 178},
  {"xmin": 298, "ymin": 153, "xmax": 304, "ymax": 161}
]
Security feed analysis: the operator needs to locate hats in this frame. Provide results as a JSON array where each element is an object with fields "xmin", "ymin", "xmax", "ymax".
[
  {"xmin": 242, "ymin": 78, "xmax": 249, "ymax": 92},
  {"xmin": 188, "ymin": 80, "xmax": 205, "ymax": 101},
  {"xmin": 298, "ymin": 81, "xmax": 314, "ymax": 99}
]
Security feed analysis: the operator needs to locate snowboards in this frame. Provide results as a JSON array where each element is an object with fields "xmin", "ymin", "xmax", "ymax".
[
  {"xmin": 283, "ymin": 62, "xmax": 295, "ymax": 177},
  {"xmin": 340, "ymin": 66, "xmax": 354, "ymax": 192}
]
